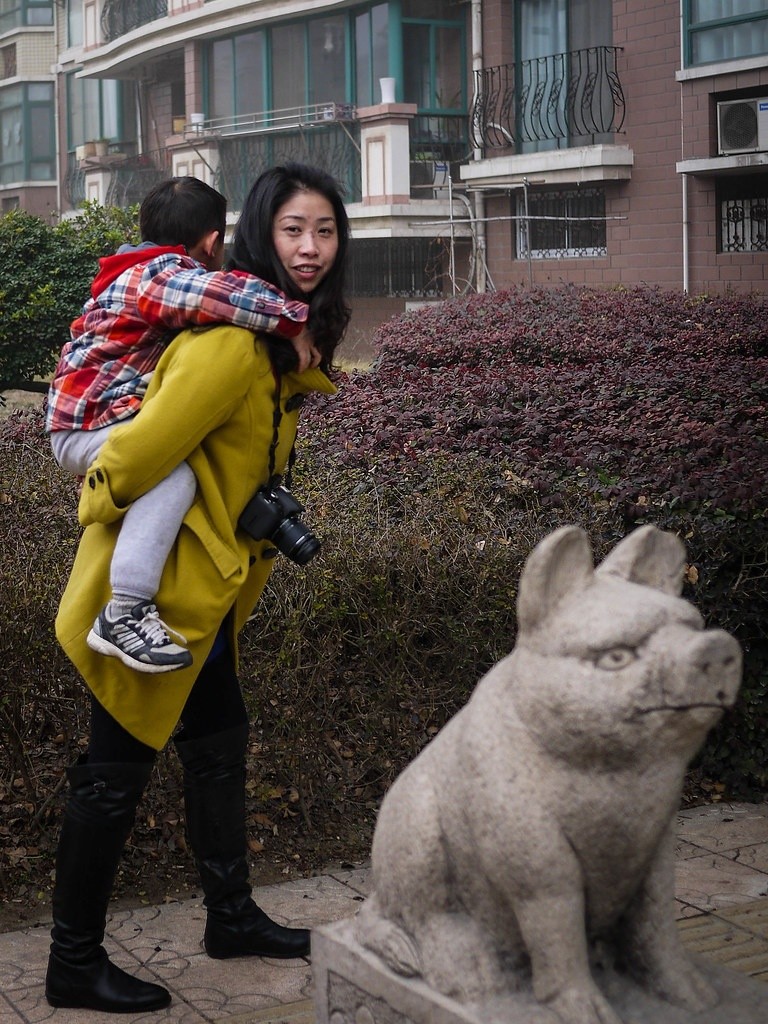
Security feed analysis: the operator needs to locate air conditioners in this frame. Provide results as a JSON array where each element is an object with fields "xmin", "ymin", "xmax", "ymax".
[
  {"xmin": 717, "ymin": 97, "xmax": 768, "ymax": 155},
  {"xmin": 410, "ymin": 161, "xmax": 451, "ymax": 200}
]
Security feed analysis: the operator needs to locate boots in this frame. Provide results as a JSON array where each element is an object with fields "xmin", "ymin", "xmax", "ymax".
[
  {"xmin": 173, "ymin": 721, "xmax": 312, "ymax": 960},
  {"xmin": 43, "ymin": 754, "xmax": 172, "ymax": 1014}
]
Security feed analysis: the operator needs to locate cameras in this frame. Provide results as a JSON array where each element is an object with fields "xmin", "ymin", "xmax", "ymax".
[{"xmin": 236, "ymin": 473, "xmax": 322, "ymax": 566}]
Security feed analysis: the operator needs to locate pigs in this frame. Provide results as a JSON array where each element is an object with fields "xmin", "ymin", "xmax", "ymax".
[{"xmin": 355, "ymin": 523, "xmax": 743, "ymax": 1023}]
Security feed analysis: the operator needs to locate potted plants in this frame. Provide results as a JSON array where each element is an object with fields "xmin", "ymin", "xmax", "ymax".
[
  {"xmin": 171, "ymin": 116, "xmax": 188, "ymax": 134},
  {"xmin": 84, "ymin": 139, "xmax": 109, "ymax": 157}
]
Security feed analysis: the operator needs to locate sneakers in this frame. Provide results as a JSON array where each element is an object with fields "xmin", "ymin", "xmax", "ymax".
[{"xmin": 87, "ymin": 600, "xmax": 193, "ymax": 672}]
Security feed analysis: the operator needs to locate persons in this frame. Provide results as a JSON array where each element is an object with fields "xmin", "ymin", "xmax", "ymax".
[
  {"xmin": 46, "ymin": 174, "xmax": 323, "ymax": 676},
  {"xmin": 45, "ymin": 158, "xmax": 358, "ymax": 1016}
]
